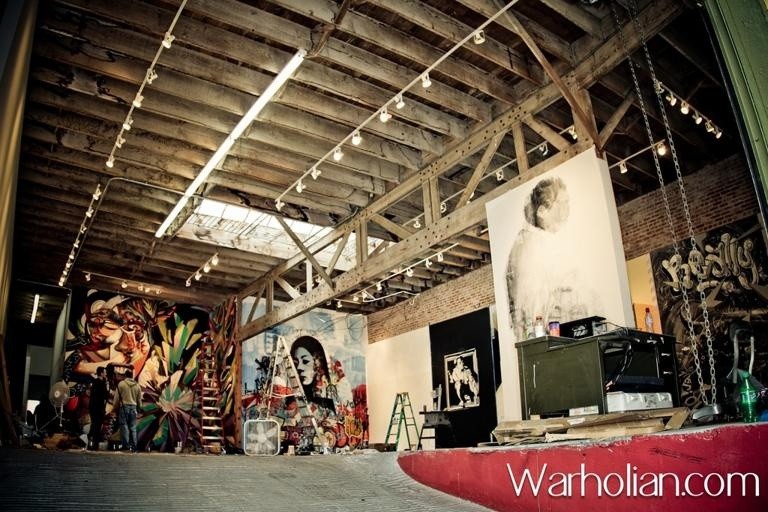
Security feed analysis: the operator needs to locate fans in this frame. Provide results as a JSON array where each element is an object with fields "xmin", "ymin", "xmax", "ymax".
[
  {"xmin": 242, "ymin": 419, "xmax": 280, "ymax": 457},
  {"xmin": 48, "ymin": 378, "xmax": 70, "ymax": 429}
]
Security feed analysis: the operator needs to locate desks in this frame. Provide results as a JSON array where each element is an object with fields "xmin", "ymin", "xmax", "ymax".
[{"xmin": 411, "ymin": 409, "xmax": 461, "ymax": 450}]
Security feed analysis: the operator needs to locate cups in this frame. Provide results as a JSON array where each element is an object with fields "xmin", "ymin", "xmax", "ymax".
[{"xmin": 549, "ymin": 322, "xmax": 561, "ymax": 337}]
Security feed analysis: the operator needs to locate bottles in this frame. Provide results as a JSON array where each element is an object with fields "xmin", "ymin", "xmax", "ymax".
[
  {"xmin": 644, "ymin": 307, "xmax": 654, "ymax": 333},
  {"xmin": 741, "ymin": 373, "xmax": 758, "ymax": 422},
  {"xmin": 535, "ymin": 315, "xmax": 548, "ymax": 337}
]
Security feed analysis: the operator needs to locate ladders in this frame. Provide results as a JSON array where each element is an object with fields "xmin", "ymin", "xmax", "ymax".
[
  {"xmin": 181, "ymin": 342, "xmax": 229, "ymax": 454},
  {"xmin": 258, "ymin": 335, "xmax": 328, "ymax": 454},
  {"xmin": 384, "ymin": 392, "xmax": 422, "ymax": 451}
]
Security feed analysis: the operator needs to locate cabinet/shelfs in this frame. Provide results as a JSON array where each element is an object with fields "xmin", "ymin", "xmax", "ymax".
[{"xmin": 514, "ymin": 329, "xmax": 682, "ymax": 422}]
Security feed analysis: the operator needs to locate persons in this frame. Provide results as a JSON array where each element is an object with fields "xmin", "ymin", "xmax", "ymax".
[
  {"xmin": 285, "ymin": 335, "xmax": 335, "ymax": 418},
  {"xmin": 112, "ymin": 370, "xmax": 143, "ymax": 452},
  {"xmin": 460, "ymin": 384, "xmax": 479, "ymax": 408},
  {"xmin": 448, "ymin": 356, "xmax": 478, "ymax": 409},
  {"xmin": 505, "ymin": 177, "xmax": 575, "ymax": 342},
  {"xmin": 87, "ymin": 366, "xmax": 115, "ymax": 451}
]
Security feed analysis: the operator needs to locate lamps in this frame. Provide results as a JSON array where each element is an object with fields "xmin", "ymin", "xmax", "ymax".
[{"xmin": 0, "ymin": 0, "xmax": 723, "ymax": 328}]
